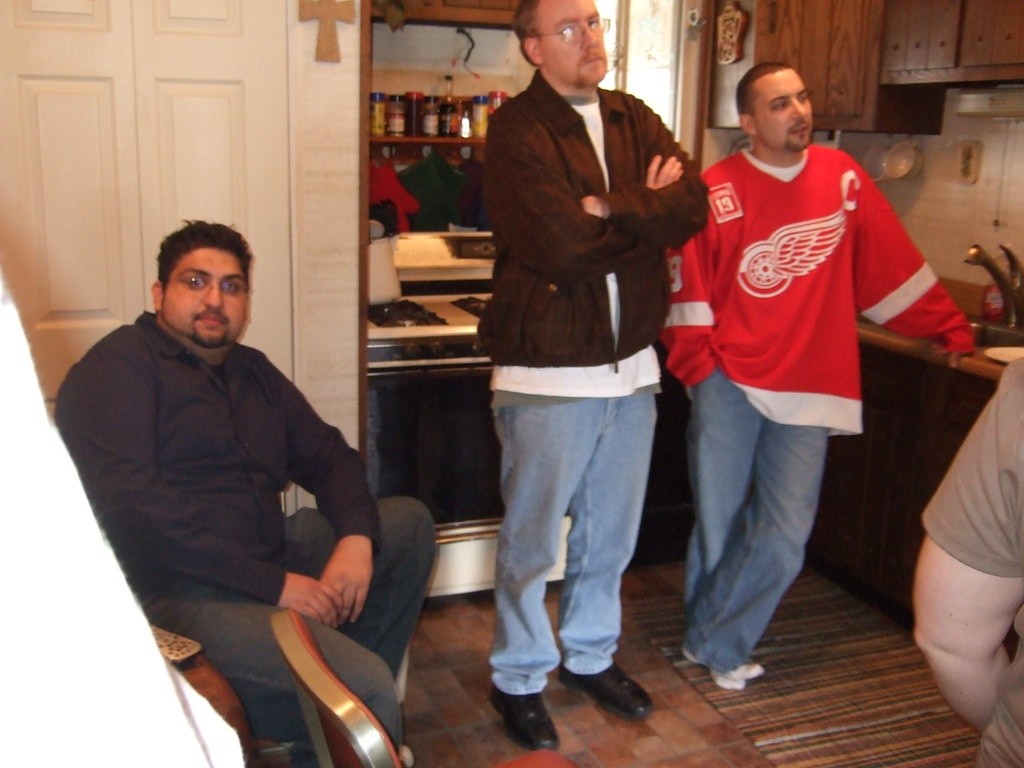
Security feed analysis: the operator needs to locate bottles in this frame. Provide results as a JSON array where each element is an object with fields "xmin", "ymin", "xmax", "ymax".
[{"xmin": 370, "ymin": 74, "xmax": 515, "ymax": 140}]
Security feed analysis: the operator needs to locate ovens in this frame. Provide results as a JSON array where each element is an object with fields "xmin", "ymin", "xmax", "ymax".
[{"xmin": 359, "ymin": 330, "xmax": 576, "ymax": 541}]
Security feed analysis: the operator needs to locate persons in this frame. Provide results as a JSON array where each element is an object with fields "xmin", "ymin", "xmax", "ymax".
[
  {"xmin": 913, "ymin": 356, "xmax": 1024, "ymax": 768},
  {"xmin": 478, "ymin": 0, "xmax": 709, "ymax": 751},
  {"xmin": 659, "ymin": 62, "xmax": 973, "ymax": 690},
  {"xmin": 55, "ymin": 221, "xmax": 437, "ymax": 768}
]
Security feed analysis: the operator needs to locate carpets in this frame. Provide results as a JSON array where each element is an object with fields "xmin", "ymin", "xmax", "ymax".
[{"xmin": 623, "ymin": 588, "xmax": 984, "ymax": 768}]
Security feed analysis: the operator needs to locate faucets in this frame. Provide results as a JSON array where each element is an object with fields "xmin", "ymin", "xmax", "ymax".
[{"xmin": 964, "ymin": 243, "xmax": 1024, "ymax": 327}]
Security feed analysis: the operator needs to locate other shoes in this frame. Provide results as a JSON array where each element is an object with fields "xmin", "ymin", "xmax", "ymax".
[
  {"xmin": 709, "ymin": 665, "xmax": 746, "ymax": 691},
  {"xmin": 681, "ymin": 646, "xmax": 764, "ymax": 680}
]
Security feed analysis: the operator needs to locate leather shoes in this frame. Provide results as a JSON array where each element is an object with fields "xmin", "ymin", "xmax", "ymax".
[
  {"xmin": 559, "ymin": 662, "xmax": 651, "ymax": 719},
  {"xmin": 489, "ymin": 684, "xmax": 559, "ymax": 749}
]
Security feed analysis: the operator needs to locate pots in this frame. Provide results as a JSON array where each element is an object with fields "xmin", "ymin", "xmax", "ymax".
[{"xmin": 862, "ymin": 136, "xmax": 924, "ymax": 183}]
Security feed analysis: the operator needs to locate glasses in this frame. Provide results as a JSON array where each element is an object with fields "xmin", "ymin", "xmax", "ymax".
[
  {"xmin": 538, "ymin": 19, "xmax": 610, "ymax": 45},
  {"xmin": 169, "ymin": 275, "xmax": 246, "ymax": 296}
]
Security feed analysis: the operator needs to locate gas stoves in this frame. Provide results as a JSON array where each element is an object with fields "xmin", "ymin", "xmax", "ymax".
[{"xmin": 363, "ymin": 223, "xmax": 502, "ymax": 348}]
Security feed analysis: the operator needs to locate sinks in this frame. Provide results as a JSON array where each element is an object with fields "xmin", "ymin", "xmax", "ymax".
[{"xmin": 970, "ymin": 323, "xmax": 1024, "ymax": 350}]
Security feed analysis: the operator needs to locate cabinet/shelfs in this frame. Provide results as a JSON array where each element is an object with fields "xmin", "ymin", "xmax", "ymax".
[
  {"xmin": 358, "ymin": 1, "xmax": 627, "ymax": 145},
  {"xmin": 804, "ymin": 343, "xmax": 1002, "ymax": 635},
  {"xmin": 707, "ymin": 1, "xmax": 1024, "ymax": 134}
]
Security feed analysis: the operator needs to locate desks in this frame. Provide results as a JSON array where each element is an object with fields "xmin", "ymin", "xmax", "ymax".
[{"xmin": 391, "ymin": 230, "xmax": 497, "ymax": 280}]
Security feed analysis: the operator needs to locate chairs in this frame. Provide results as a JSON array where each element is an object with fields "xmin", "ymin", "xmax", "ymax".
[{"xmin": 268, "ymin": 607, "xmax": 582, "ymax": 767}]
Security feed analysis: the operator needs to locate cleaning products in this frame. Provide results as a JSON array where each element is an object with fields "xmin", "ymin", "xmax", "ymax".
[{"xmin": 981, "ymin": 241, "xmax": 1014, "ymax": 322}]
